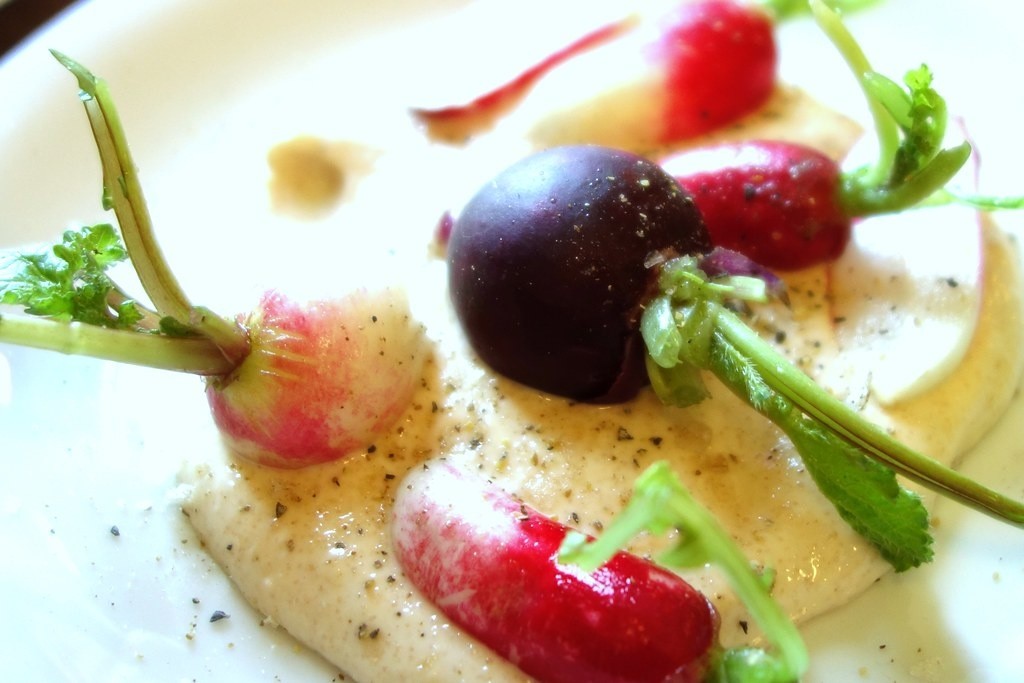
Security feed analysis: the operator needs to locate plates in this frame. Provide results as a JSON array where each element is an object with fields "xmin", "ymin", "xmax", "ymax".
[{"xmin": 0, "ymin": 0, "xmax": 1024, "ymax": 683}]
